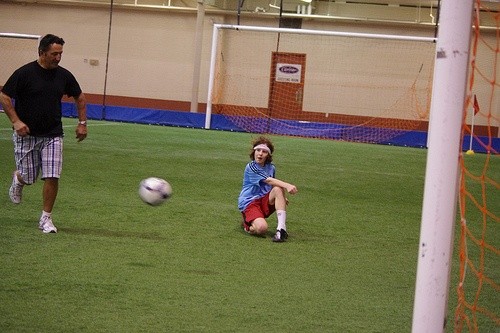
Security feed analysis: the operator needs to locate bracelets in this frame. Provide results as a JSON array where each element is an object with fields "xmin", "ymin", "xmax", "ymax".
[{"xmin": 78, "ymin": 120, "xmax": 86, "ymax": 126}]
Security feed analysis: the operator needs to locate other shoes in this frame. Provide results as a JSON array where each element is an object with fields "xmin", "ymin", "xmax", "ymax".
[{"xmin": 272, "ymin": 228, "xmax": 288, "ymax": 242}]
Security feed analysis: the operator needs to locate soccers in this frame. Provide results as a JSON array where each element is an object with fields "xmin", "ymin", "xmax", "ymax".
[{"xmin": 137, "ymin": 177, "xmax": 174, "ymax": 207}]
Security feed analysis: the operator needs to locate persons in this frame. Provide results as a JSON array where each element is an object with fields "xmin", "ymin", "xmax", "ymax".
[
  {"xmin": 0, "ymin": 34, "xmax": 88, "ymax": 233},
  {"xmin": 238, "ymin": 136, "xmax": 298, "ymax": 242}
]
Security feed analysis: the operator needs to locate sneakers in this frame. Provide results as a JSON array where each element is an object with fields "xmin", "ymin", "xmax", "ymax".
[
  {"xmin": 38, "ymin": 215, "xmax": 57, "ymax": 233},
  {"xmin": 9, "ymin": 171, "xmax": 23, "ymax": 203}
]
